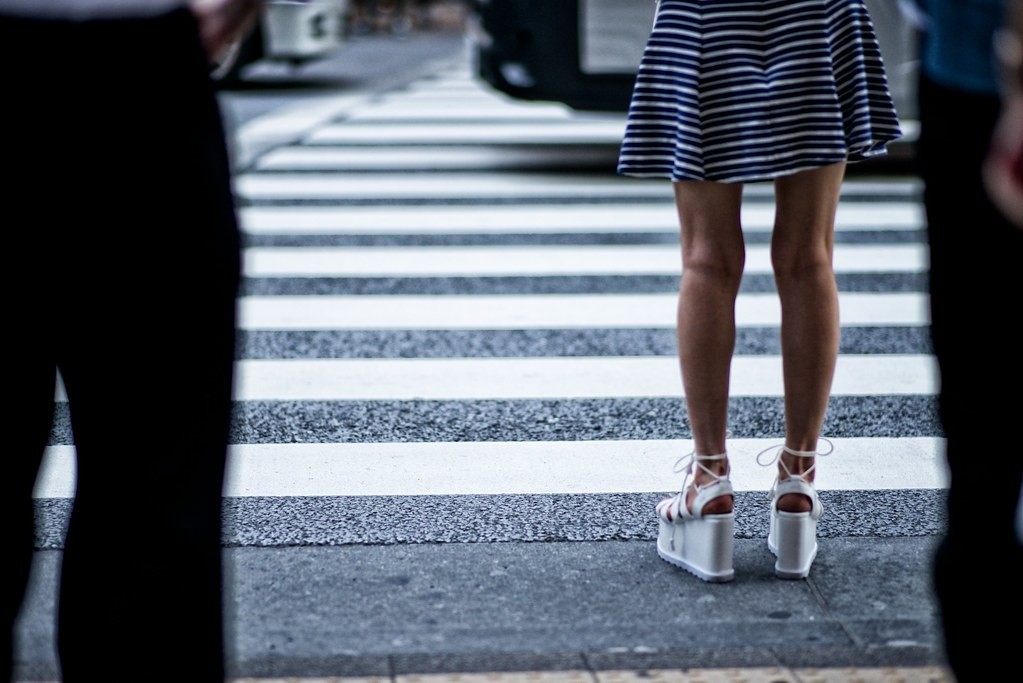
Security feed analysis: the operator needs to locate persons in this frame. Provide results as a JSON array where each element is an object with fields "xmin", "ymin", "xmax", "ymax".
[
  {"xmin": 614, "ymin": 0, "xmax": 903, "ymax": 582},
  {"xmin": 894, "ymin": 0, "xmax": 1023, "ymax": 683},
  {"xmin": 0, "ymin": 1, "xmax": 302, "ymax": 683}
]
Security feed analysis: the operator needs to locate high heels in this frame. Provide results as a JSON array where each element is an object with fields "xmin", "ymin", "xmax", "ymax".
[
  {"xmin": 757, "ymin": 436, "xmax": 835, "ymax": 578},
  {"xmin": 657, "ymin": 450, "xmax": 736, "ymax": 583}
]
